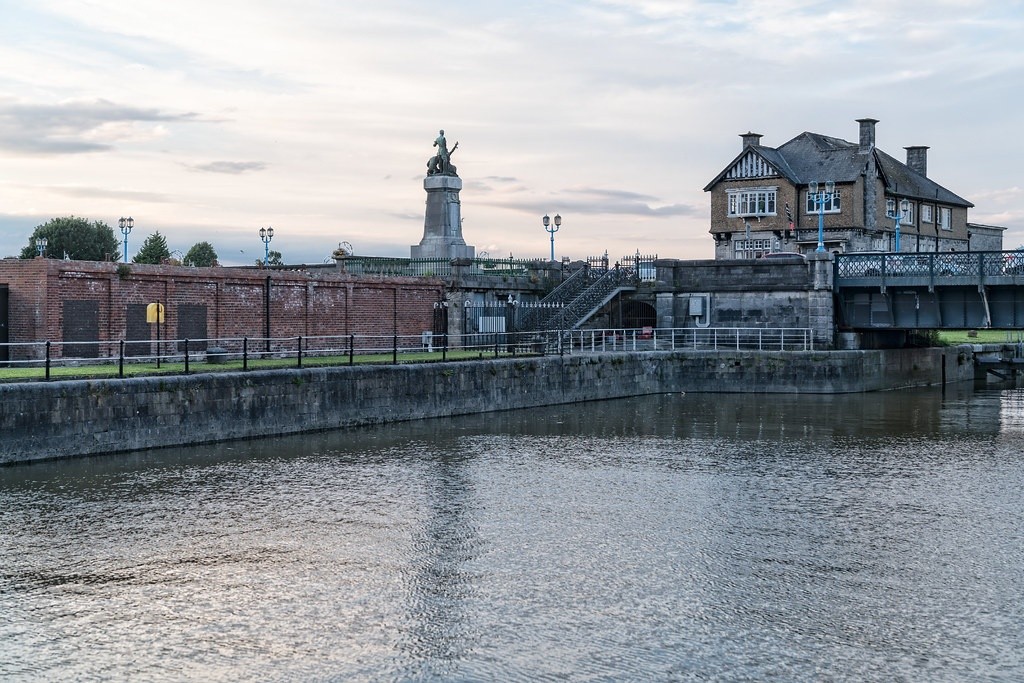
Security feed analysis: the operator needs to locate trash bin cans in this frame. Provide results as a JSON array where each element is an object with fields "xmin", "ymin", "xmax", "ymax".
[
  {"xmin": 531, "ymin": 336, "xmax": 545, "ymax": 353},
  {"xmin": 206, "ymin": 347, "xmax": 227, "ymax": 363}
]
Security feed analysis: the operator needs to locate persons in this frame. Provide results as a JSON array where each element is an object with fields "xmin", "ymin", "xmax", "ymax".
[{"xmin": 427, "ymin": 130, "xmax": 459, "ymax": 174}]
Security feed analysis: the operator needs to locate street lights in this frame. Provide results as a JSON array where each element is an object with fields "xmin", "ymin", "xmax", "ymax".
[
  {"xmin": 119, "ymin": 216, "xmax": 133, "ymax": 263},
  {"xmin": 543, "ymin": 212, "xmax": 562, "ymax": 265},
  {"xmin": 259, "ymin": 225, "xmax": 273, "ymax": 266},
  {"xmin": 886, "ymin": 197, "xmax": 909, "ymax": 252},
  {"xmin": 809, "ymin": 179, "xmax": 834, "ymax": 252}
]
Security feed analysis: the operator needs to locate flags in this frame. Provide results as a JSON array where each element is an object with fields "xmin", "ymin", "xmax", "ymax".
[{"xmin": 785, "ymin": 199, "xmax": 794, "ymax": 230}]
